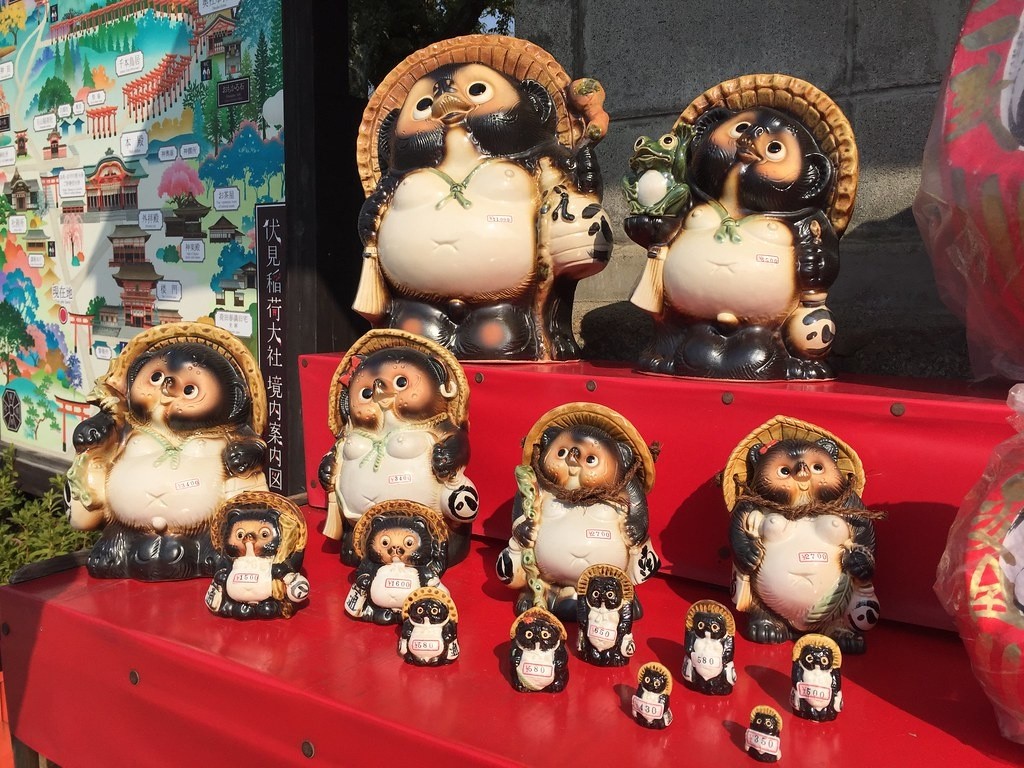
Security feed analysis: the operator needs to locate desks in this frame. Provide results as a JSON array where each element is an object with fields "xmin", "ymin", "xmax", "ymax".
[{"xmin": 1, "ymin": 351, "xmax": 1024, "ymax": 768}]
